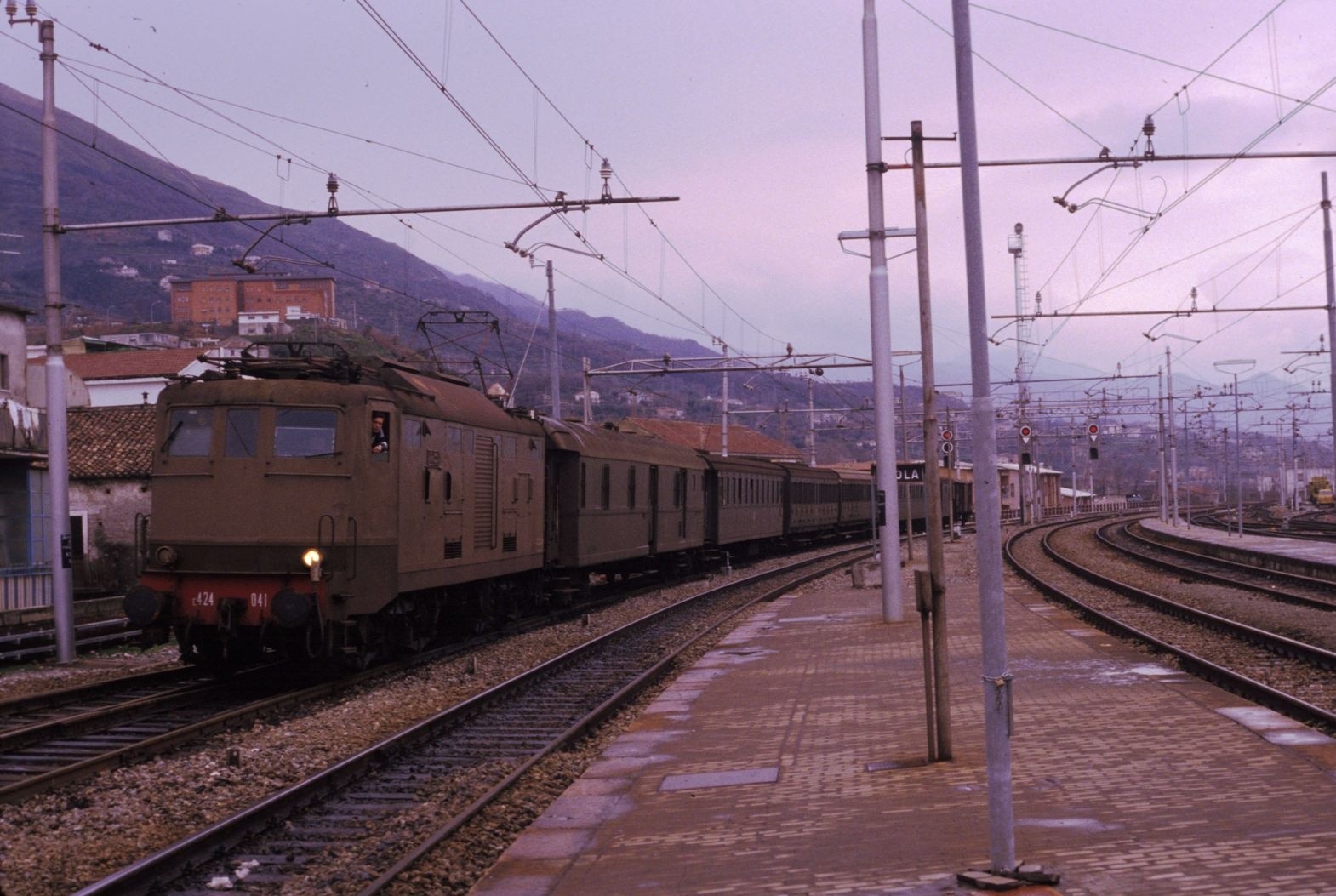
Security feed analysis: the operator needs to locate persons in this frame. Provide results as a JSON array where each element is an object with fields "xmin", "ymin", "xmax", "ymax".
[{"xmin": 371, "ymin": 413, "xmax": 388, "ymax": 453}]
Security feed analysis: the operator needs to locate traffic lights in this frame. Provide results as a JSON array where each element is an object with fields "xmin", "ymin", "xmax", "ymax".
[
  {"xmin": 1089, "ymin": 423, "xmax": 1100, "ymax": 435},
  {"xmin": 940, "ymin": 429, "xmax": 954, "ymax": 454},
  {"xmin": 1020, "ymin": 426, "xmax": 1032, "ymax": 438}
]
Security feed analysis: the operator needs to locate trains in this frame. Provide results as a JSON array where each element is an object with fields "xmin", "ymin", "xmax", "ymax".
[
  {"xmin": 1307, "ymin": 476, "xmax": 1335, "ymax": 506},
  {"xmin": 123, "ymin": 309, "xmax": 973, "ymax": 691}
]
